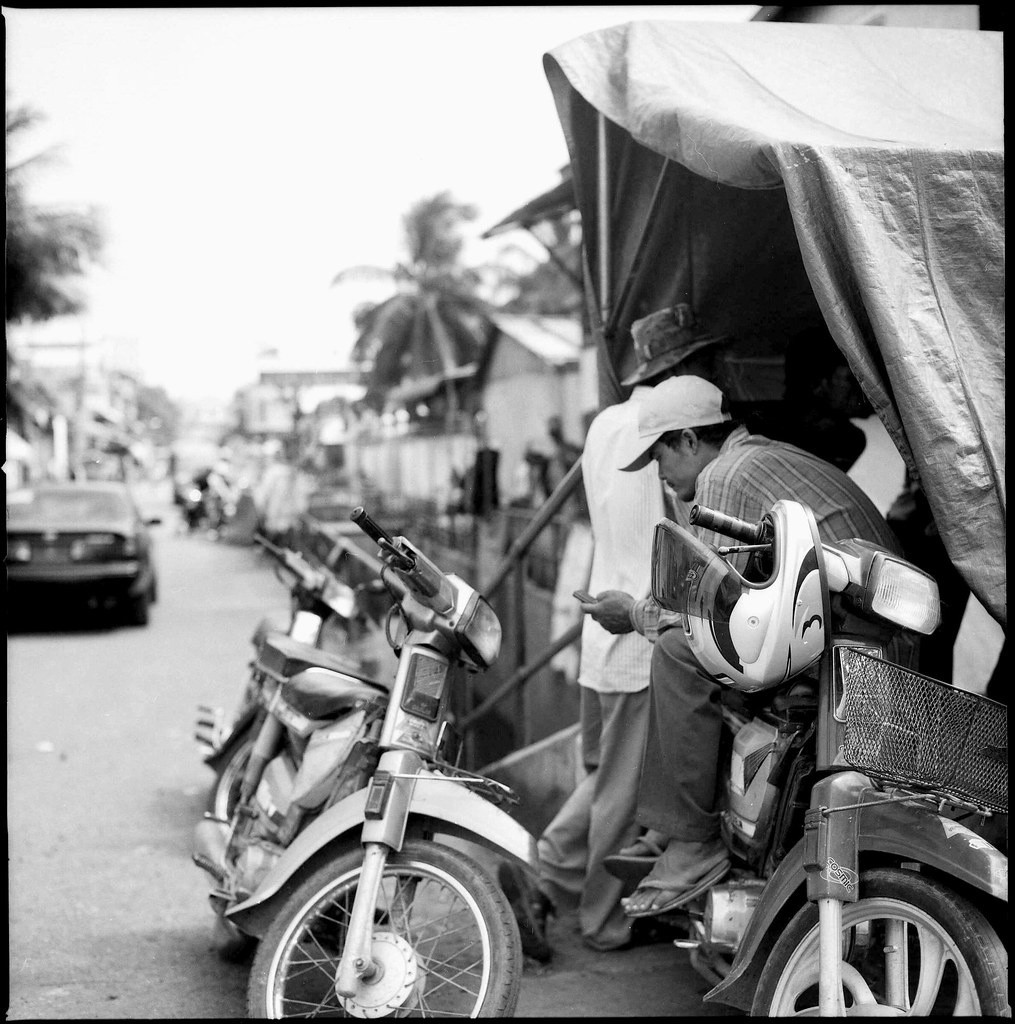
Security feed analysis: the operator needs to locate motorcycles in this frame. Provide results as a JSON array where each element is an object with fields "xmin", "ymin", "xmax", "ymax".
[
  {"xmin": 193, "ymin": 506, "xmax": 542, "ymax": 1019},
  {"xmin": 654, "ymin": 503, "xmax": 1012, "ymax": 1017}
]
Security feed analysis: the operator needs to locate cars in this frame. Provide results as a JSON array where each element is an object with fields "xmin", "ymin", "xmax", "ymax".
[{"xmin": 7, "ymin": 481, "xmax": 160, "ymax": 626}]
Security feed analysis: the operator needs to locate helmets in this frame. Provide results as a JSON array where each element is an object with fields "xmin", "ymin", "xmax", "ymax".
[{"xmin": 651, "ymin": 498, "xmax": 832, "ymax": 695}]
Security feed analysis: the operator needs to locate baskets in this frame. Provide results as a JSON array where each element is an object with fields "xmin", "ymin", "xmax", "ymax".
[{"xmin": 839, "ymin": 645, "xmax": 1008, "ymax": 813}]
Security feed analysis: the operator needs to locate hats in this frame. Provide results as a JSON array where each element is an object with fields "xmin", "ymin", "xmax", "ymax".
[
  {"xmin": 618, "ymin": 375, "xmax": 731, "ymax": 471},
  {"xmin": 620, "ymin": 303, "xmax": 730, "ymax": 387}
]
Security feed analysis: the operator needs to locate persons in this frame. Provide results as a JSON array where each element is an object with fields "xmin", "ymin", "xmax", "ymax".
[
  {"xmin": 498, "ymin": 303, "xmax": 723, "ymax": 963},
  {"xmin": 580, "ymin": 373, "xmax": 921, "ymax": 918}
]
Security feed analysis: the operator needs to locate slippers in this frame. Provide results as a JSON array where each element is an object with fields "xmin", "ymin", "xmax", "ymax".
[
  {"xmin": 627, "ymin": 852, "xmax": 732, "ymax": 918},
  {"xmin": 497, "ymin": 859, "xmax": 553, "ymax": 964},
  {"xmin": 604, "ymin": 838, "xmax": 663, "ymax": 885},
  {"xmin": 613, "ymin": 920, "xmax": 691, "ymax": 954}
]
